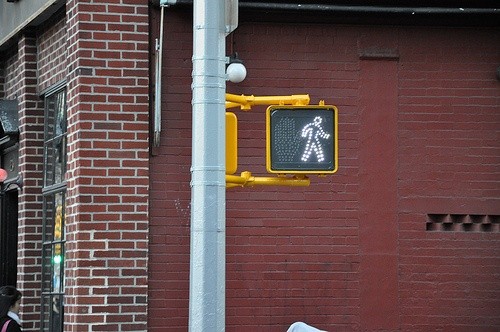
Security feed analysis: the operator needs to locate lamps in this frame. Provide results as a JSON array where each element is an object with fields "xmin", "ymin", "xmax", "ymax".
[{"xmin": 228, "ymin": 51, "xmax": 247, "ymax": 84}]
